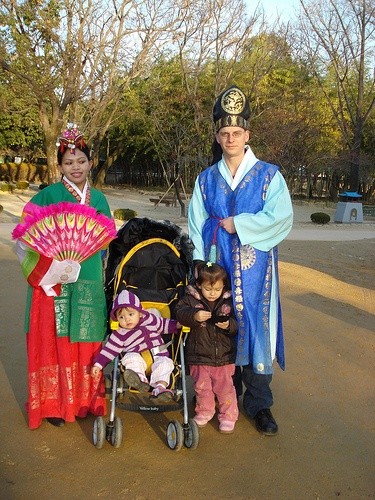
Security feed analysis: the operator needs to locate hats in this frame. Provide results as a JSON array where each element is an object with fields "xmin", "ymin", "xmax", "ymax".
[
  {"xmin": 213, "ymin": 85, "xmax": 251, "ymax": 131},
  {"xmin": 109, "ymin": 290, "xmax": 142, "ymax": 321}
]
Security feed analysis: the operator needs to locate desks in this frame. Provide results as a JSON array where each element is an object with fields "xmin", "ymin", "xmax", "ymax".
[{"xmin": 159, "ymin": 195, "xmax": 188, "ymax": 207}]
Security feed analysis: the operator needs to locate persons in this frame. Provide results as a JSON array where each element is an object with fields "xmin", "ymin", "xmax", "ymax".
[
  {"xmin": 175, "ymin": 261, "xmax": 239, "ymax": 434},
  {"xmin": 186, "ymin": 85, "xmax": 294, "ymax": 435},
  {"xmin": 89, "ymin": 291, "xmax": 183, "ymax": 404},
  {"xmin": 15, "ymin": 123, "xmax": 115, "ymax": 430}
]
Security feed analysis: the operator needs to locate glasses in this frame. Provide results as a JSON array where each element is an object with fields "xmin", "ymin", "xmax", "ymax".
[{"xmin": 220, "ymin": 131, "xmax": 244, "ymax": 139}]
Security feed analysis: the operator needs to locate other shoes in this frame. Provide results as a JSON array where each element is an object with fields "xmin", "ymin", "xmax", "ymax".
[
  {"xmin": 193, "ymin": 414, "xmax": 214, "ymax": 428},
  {"xmin": 123, "ymin": 369, "xmax": 150, "ymax": 393},
  {"xmin": 46, "ymin": 418, "xmax": 65, "ymax": 427},
  {"xmin": 219, "ymin": 420, "xmax": 235, "ymax": 434},
  {"xmin": 149, "ymin": 387, "xmax": 174, "ymax": 403},
  {"xmin": 253, "ymin": 408, "xmax": 279, "ymax": 436}
]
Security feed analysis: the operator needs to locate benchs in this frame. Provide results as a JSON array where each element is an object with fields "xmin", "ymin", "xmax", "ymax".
[{"xmin": 149, "ymin": 198, "xmax": 173, "ymax": 207}]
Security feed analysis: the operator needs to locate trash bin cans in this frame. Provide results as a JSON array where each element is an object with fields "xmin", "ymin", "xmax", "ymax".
[{"xmin": 333, "ymin": 191, "xmax": 363, "ymax": 223}]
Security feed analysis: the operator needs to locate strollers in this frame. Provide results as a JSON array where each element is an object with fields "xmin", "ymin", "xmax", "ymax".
[{"xmin": 92, "ymin": 216, "xmax": 201, "ymax": 452}]
